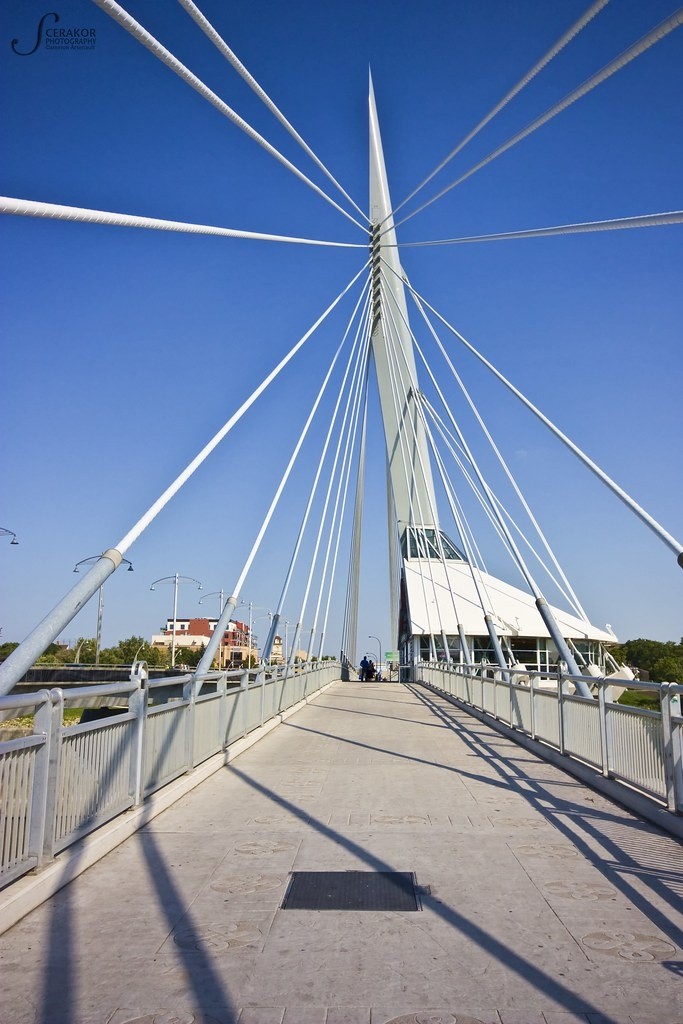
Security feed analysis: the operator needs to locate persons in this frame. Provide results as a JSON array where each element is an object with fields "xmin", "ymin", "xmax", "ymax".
[
  {"xmin": 239, "ymin": 663, "xmax": 243, "ymax": 670},
  {"xmin": 359, "ymin": 656, "xmax": 387, "ymax": 682},
  {"xmin": 180, "ymin": 662, "xmax": 187, "ymax": 671}
]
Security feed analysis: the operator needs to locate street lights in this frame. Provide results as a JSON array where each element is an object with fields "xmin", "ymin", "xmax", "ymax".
[
  {"xmin": 368, "ymin": 635, "xmax": 381, "ymax": 674},
  {"xmin": 235, "ymin": 602, "xmax": 272, "ymax": 669},
  {"xmin": 365, "ymin": 651, "xmax": 377, "ymax": 671},
  {"xmin": 74, "ymin": 551, "xmax": 134, "ymax": 667},
  {"xmin": 199, "ymin": 588, "xmax": 245, "ymax": 673},
  {"xmin": 149, "ymin": 574, "xmax": 203, "ymax": 668},
  {"xmin": 251, "ymin": 613, "xmax": 317, "ymax": 667}
]
